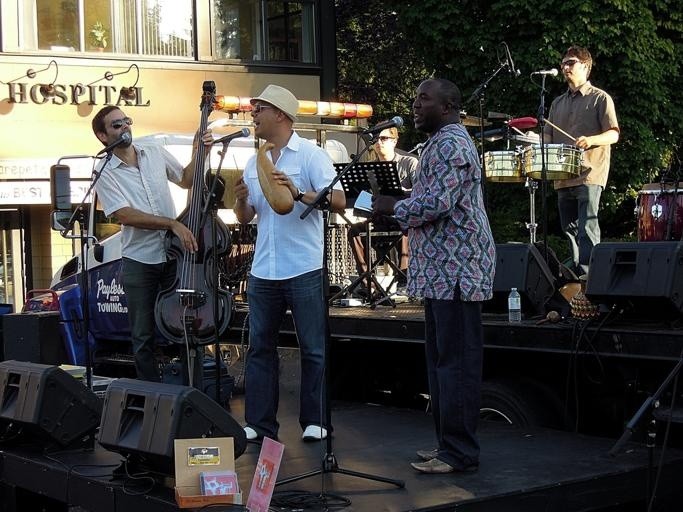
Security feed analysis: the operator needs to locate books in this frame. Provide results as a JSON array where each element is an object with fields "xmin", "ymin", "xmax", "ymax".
[{"xmin": 197, "ymin": 470, "xmax": 239, "ymax": 495}]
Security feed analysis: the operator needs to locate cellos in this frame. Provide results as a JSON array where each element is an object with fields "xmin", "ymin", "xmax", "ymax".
[{"xmin": 154, "ymin": 82, "xmax": 235, "ymax": 344}]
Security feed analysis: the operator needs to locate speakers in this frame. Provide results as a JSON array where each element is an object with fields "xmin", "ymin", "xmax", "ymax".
[
  {"xmin": 584, "ymin": 241, "xmax": 683, "ymax": 326},
  {"xmin": 96, "ymin": 377, "xmax": 247, "ymax": 481},
  {"xmin": 483, "ymin": 242, "xmax": 557, "ymax": 315},
  {"xmin": 0, "ymin": 360, "xmax": 102, "ymax": 450}
]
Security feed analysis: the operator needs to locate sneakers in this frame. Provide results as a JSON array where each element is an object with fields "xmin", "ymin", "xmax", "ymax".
[
  {"xmin": 301, "ymin": 425, "xmax": 328, "ymax": 443},
  {"xmin": 242, "ymin": 427, "xmax": 258, "ymax": 439}
]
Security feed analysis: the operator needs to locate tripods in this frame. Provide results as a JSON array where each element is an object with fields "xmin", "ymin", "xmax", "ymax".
[{"xmin": 276, "ymin": 220, "xmax": 405, "ymax": 491}]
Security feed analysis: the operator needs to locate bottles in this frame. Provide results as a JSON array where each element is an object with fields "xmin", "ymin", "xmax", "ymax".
[{"xmin": 508, "ymin": 288, "xmax": 521, "ymax": 323}]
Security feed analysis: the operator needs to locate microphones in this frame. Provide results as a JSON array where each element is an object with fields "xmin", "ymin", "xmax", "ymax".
[
  {"xmin": 97, "ymin": 132, "xmax": 132, "ymax": 154},
  {"xmin": 216, "ymin": 128, "xmax": 252, "ymax": 143},
  {"xmin": 535, "ymin": 68, "xmax": 559, "ymax": 78},
  {"xmin": 362, "ymin": 117, "xmax": 405, "ymax": 134}
]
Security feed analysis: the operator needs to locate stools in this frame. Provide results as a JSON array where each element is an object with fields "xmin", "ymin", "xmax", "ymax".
[{"xmin": 358, "ymin": 232, "xmax": 407, "ymax": 307}]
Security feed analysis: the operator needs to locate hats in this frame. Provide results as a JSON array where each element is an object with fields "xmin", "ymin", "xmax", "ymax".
[{"xmin": 250, "ymin": 84, "xmax": 300, "ymax": 123}]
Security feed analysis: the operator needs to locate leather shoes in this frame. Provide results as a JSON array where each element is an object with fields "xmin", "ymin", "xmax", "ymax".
[
  {"xmin": 415, "ymin": 448, "xmax": 439, "ymax": 461},
  {"xmin": 410, "ymin": 457, "xmax": 455, "ymax": 473}
]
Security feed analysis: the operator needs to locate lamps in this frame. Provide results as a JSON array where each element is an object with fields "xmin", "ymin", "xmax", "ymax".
[
  {"xmin": 27, "ymin": 60, "xmax": 58, "ymax": 98},
  {"xmin": 105, "ymin": 64, "xmax": 139, "ymax": 100}
]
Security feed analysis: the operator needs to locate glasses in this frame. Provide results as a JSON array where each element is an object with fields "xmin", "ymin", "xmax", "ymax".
[
  {"xmin": 111, "ymin": 117, "xmax": 133, "ymax": 129},
  {"xmin": 560, "ymin": 60, "xmax": 583, "ymax": 70},
  {"xmin": 378, "ymin": 136, "xmax": 395, "ymax": 142},
  {"xmin": 253, "ymin": 104, "xmax": 271, "ymax": 114}
]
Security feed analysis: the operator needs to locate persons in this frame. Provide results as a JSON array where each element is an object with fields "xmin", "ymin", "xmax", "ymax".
[
  {"xmin": 91, "ymin": 104, "xmax": 214, "ymax": 383},
  {"xmin": 371, "ymin": 78, "xmax": 498, "ymax": 475},
  {"xmin": 230, "ymin": 84, "xmax": 347, "ymax": 443},
  {"xmin": 524, "ymin": 44, "xmax": 621, "ymax": 277},
  {"xmin": 347, "ymin": 120, "xmax": 419, "ymax": 303}
]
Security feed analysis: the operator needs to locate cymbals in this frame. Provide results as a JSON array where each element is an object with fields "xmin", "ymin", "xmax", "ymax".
[{"xmin": 459, "ymin": 112, "xmax": 492, "ymax": 128}]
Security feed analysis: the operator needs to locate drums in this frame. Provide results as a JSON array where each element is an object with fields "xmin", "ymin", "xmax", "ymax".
[
  {"xmin": 481, "ymin": 151, "xmax": 521, "ymax": 184},
  {"xmin": 635, "ymin": 188, "xmax": 682, "ymax": 241},
  {"xmin": 520, "ymin": 144, "xmax": 580, "ymax": 181}
]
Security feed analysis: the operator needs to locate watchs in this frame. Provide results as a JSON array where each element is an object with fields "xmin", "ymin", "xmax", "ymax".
[{"xmin": 295, "ymin": 186, "xmax": 306, "ymax": 202}]
